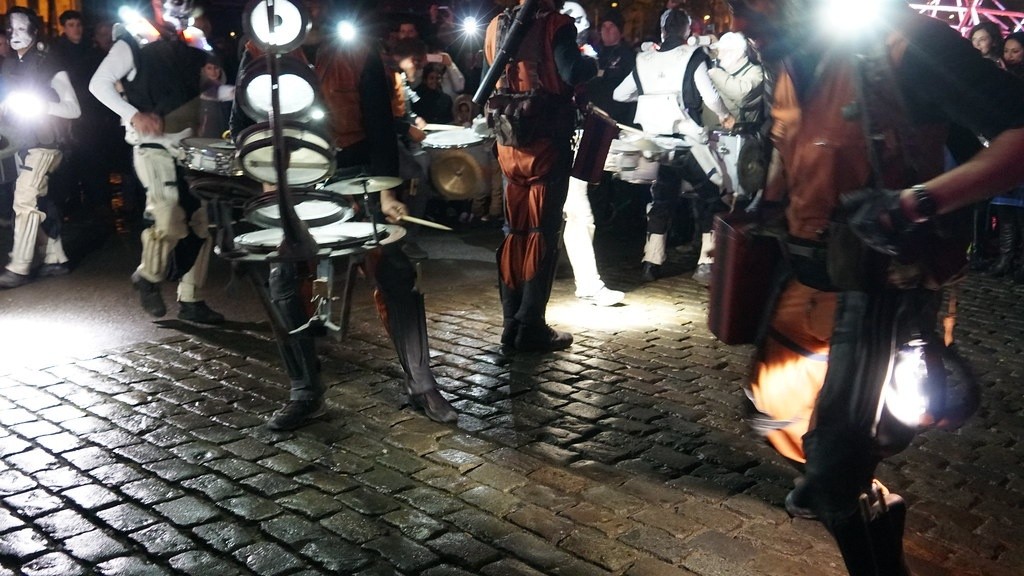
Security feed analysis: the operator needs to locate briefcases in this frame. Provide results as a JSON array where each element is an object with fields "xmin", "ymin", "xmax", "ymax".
[{"xmin": 707, "ymin": 213, "xmax": 780, "ymax": 344}]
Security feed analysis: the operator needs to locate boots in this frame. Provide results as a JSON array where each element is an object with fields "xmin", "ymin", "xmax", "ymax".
[
  {"xmin": 989, "ymin": 207, "xmax": 1017, "ymax": 277},
  {"xmin": 1017, "ymin": 264, "xmax": 1024, "ymax": 284}
]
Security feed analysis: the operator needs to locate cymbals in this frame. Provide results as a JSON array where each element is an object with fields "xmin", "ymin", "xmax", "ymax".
[
  {"xmin": 323, "ymin": 175, "xmax": 404, "ymax": 194},
  {"xmin": 429, "ymin": 150, "xmax": 482, "ymax": 199}
]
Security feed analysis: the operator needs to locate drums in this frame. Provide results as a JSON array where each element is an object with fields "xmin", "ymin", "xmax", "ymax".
[
  {"xmin": 710, "ymin": 129, "xmax": 752, "ymax": 201},
  {"xmin": 241, "ymin": 0, "xmax": 311, "ymax": 54},
  {"xmin": 573, "ymin": 129, "xmax": 641, "ymax": 172},
  {"xmin": 236, "ymin": 55, "xmax": 319, "ymax": 124},
  {"xmin": 232, "ymin": 220, "xmax": 387, "ymax": 251},
  {"xmin": 244, "ymin": 185, "xmax": 355, "ymax": 230},
  {"xmin": 421, "ymin": 126, "xmax": 491, "ymax": 198},
  {"xmin": 614, "ymin": 140, "xmax": 660, "ymax": 184},
  {"xmin": 177, "ymin": 136, "xmax": 247, "ymax": 176},
  {"xmin": 650, "ymin": 134, "xmax": 693, "ymax": 167},
  {"xmin": 236, "ymin": 120, "xmax": 337, "ymax": 188}
]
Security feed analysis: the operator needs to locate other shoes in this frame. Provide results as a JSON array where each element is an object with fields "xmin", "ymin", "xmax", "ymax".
[
  {"xmin": 471, "ymin": 217, "xmax": 483, "ymax": 228},
  {"xmin": 33, "ymin": 260, "xmax": 70, "ymax": 276},
  {"xmin": 408, "ymin": 387, "xmax": 458, "ymax": 423},
  {"xmin": 178, "ymin": 303, "xmax": 225, "ymax": 324},
  {"xmin": 130, "ymin": 269, "xmax": 166, "ymax": 317},
  {"xmin": 663, "ymin": 246, "xmax": 701, "ymax": 271},
  {"xmin": 267, "ymin": 400, "xmax": 327, "ymax": 427},
  {"xmin": 1, "ymin": 270, "xmax": 33, "ymax": 286},
  {"xmin": 573, "ymin": 288, "xmax": 593, "ymax": 299},
  {"xmin": 640, "ymin": 264, "xmax": 666, "ymax": 281},
  {"xmin": 400, "ymin": 241, "xmax": 428, "ymax": 260},
  {"xmin": 784, "ymin": 479, "xmax": 821, "ymax": 518},
  {"xmin": 693, "ymin": 265, "xmax": 715, "ymax": 286},
  {"xmin": 584, "ymin": 288, "xmax": 625, "ymax": 306},
  {"xmin": 487, "ymin": 219, "xmax": 502, "ymax": 228},
  {"xmin": 500, "ymin": 328, "xmax": 518, "ymax": 344},
  {"xmin": 514, "ymin": 325, "xmax": 573, "ymax": 351}
]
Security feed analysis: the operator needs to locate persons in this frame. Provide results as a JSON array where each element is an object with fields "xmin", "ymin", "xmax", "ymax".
[
  {"xmin": 930, "ymin": 20, "xmax": 1024, "ymax": 354},
  {"xmin": 725, "ymin": 0, "xmax": 1024, "ymax": 576},
  {"xmin": 0, "ymin": 0, "xmax": 764, "ymax": 352},
  {"xmin": 229, "ymin": 0, "xmax": 459, "ymax": 430}
]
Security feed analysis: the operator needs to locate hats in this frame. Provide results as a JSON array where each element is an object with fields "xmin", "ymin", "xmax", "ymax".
[{"xmin": 600, "ymin": 10, "xmax": 625, "ymax": 35}]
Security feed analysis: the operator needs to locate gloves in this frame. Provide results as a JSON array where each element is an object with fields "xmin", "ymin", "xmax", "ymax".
[{"xmin": 840, "ymin": 185, "xmax": 919, "ymax": 257}]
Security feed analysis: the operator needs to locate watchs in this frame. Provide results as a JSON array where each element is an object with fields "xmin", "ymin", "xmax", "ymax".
[{"xmin": 911, "ymin": 184, "xmax": 935, "ymax": 220}]
